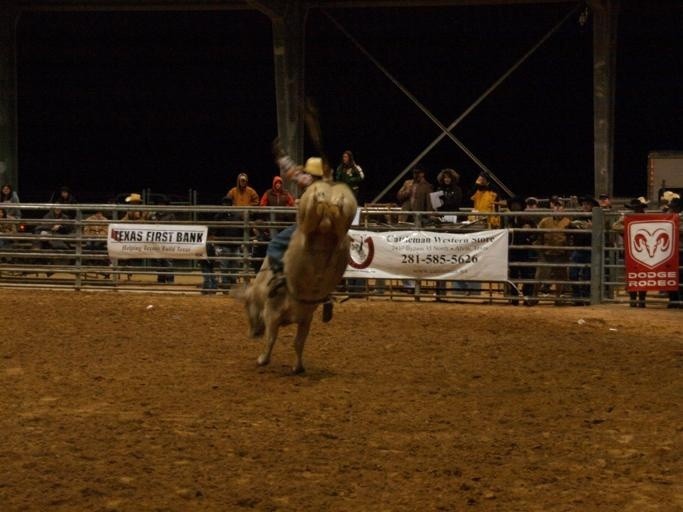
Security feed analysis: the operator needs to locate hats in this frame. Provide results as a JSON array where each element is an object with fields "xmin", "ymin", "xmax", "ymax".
[
  {"xmin": 659, "ymin": 189, "xmax": 681, "ymax": 202},
  {"xmin": 666, "ymin": 197, "xmax": 683, "ymax": 214},
  {"xmin": 636, "ymin": 196, "xmax": 651, "ymax": 205},
  {"xmin": 597, "ymin": 192, "xmax": 609, "ymax": 199},
  {"xmin": 301, "ymin": 156, "xmax": 335, "ymax": 177},
  {"xmin": 623, "ymin": 199, "xmax": 649, "ymax": 210},
  {"xmin": 547, "ymin": 195, "xmax": 564, "ymax": 206},
  {"xmin": 524, "ymin": 196, "xmax": 539, "ymax": 206},
  {"xmin": 123, "ymin": 192, "xmax": 144, "ymax": 204},
  {"xmin": 576, "ymin": 193, "xmax": 601, "ymax": 210}
]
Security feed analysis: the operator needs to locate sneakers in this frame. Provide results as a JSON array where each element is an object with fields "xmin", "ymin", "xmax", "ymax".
[
  {"xmin": 266, "ymin": 272, "xmax": 287, "ymax": 299},
  {"xmin": 629, "ymin": 298, "xmax": 647, "ymax": 308},
  {"xmin": 321, "ymin": 299, "xmax": 335, "ymax": 323}
]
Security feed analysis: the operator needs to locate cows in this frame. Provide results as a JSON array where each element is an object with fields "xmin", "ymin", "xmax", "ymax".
[{"xmin": 229, "ymin": 96, "xmax": 357, "ymax": 374}]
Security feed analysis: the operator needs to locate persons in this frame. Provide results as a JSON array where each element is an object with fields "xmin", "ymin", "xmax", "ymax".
[
  {"xmin": 613, "ymin": 190, "xmax": 683, "ymax": 310},
  {"xmin": 265, "ymin": 140, "xmax": 335, "ymax": 322},
  {"xmin": 398, "ymin": 167, "xmax": 619, "ymax": 307},
  {"xmin": 214, "ymin": 151, "xmax": 366, "ymax": 298},
  {"xmin": 0, "ymin": 184, "xmax": 175, "ymax": 284}
]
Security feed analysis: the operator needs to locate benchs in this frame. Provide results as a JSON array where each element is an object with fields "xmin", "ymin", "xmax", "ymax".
[{"xmin": 365, "ymin": 196, "xmax": 648, "ymax": 293}]
